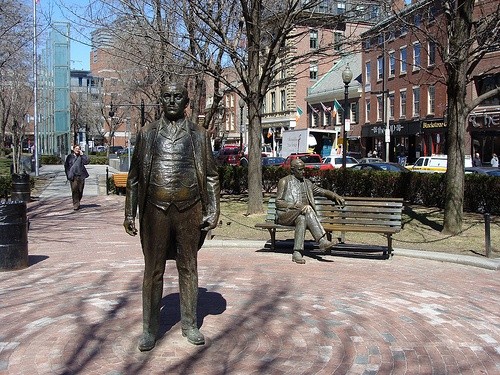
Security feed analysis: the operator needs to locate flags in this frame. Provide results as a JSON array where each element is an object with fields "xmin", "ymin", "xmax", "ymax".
[
  {"xmin": 267, "ymin": 127, "xmax": 273, "ymax": 138},
  {"xmin": 296, "ymin": 101, "xmax": 341, "ymax": 122}
]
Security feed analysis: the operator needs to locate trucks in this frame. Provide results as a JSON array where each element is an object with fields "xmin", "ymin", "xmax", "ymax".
[{"xmin": 275, "ymin": 128, "xmax": 338, "ymax": 160}]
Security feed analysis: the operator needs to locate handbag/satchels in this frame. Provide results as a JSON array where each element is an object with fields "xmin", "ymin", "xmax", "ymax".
[{"xmin": 67, "ymin": 171, "xmax": 73, "ymax": 179}]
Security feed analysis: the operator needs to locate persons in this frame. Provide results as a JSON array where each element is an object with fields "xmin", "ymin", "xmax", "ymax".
[
  {"xmin": 472, "ymin": 153, "xmax": 482, "ymax": 167},
  {"xmin": 65, "ymin": 145, "xmax": 91, "ymax": 210},
  {"xmin": 395, "ymin": 143, "xmax": 407, "ymax": 166},
  {"xmin": 123, "ymin": 84, "xmax": 221, "ymax": 352},
  {"xmin": 276, "ymin": 159, "xmax": 346, "ymax": 264},
  {"xmin": 367, "ymin": 147, "xmax": 379, "ymax": 158},
  {"xmin": 491, "ymin": 153, "xmax": 498, "ymax": 168}
]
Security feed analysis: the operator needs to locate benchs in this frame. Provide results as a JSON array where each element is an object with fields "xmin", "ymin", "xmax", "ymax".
[
  {"xmin": 113, "ymin": 174, "xmax": 127, "ymax": 196},
  {"xmin": 255, "ymin": 194, "xmax": 404, "ymax": 260}
]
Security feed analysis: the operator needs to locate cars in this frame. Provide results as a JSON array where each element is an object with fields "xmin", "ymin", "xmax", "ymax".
[
  {"xmin": 211, "ymin": 144, "xmax": 499, "ymax": 176},
  {"xmin": 88, "ymin": 146, "xmax": 135, "ymax": 155}
]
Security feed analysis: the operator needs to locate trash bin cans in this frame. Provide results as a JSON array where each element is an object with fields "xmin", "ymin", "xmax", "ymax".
[
  {"xmin": 19, "ymin": 155, "xmax": 32, "ymax": 174},
  {"xmin": 0, "ymin": 201, "xmax": 29, "ymax": 272},
  {"xmin": 11, "ymin": 169, "xmax": 31, "ymax": 203},
  {"xmin": 39, "ymin": 159, "xmax": 42, "ymax": 168},
  {"xmin": 10, "ymin": 163, "xmax": 17, "ymax": 174},
  {"xmin": 32, "ymin": 159, "xmax": 36, "ymax": 172}
]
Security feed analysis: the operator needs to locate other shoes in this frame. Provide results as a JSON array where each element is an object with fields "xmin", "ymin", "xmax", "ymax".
[{"xmin": 74, "ymin": 206, "xmax": 78, "ymax": 210}]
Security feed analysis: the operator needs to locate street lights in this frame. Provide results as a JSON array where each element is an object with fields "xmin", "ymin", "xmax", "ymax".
[
  {"xmin": 108, "ymin": 99, "xmax": 162, "ymax": 127},
  {"xmin": 238, "ymin": 96, "xmax": 246, "ymax": 160},
  {"xmin": 341, "ymin": 61, "xmax": 353, "ymax": 172}
]
